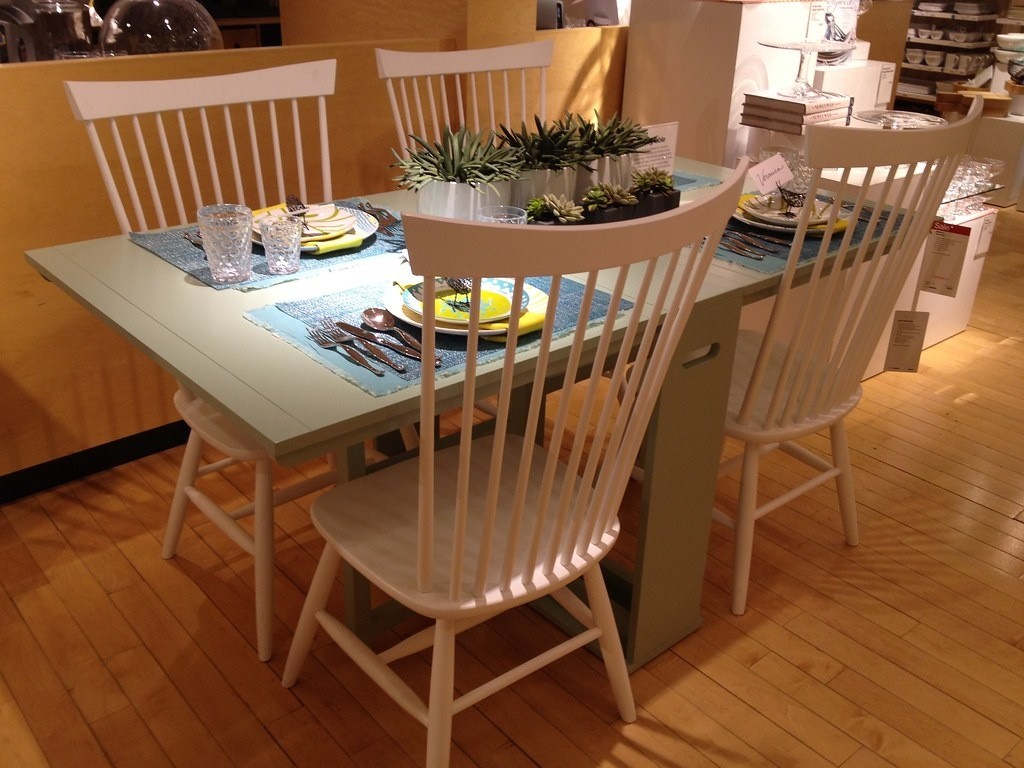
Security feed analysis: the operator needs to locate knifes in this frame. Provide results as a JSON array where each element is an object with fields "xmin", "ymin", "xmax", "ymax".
[
  {"xmin": 335, "ymin": 321, "xmax": 442, "ymax": 368},
  {"xmin": 376, "ymin": 226, "xmax": 395, "ymax": 237}
]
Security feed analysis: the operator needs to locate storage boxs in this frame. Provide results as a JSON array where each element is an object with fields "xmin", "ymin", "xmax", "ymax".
[{"xmin": 935, "ymin": 78, "xmax": 1024, "ymax": 117}]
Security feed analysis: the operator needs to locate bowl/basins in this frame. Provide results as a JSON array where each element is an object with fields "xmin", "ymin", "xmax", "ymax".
[
  {"xmin": 904, "ymin": 25, "xmax": 945, "ymax": 65},
  {"xmin": 991, "ymin": 32, "xmax": 1024, "ymax": 66}
]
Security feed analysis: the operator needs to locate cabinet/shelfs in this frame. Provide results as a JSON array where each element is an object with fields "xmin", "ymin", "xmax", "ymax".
[{"xmin": 894, "ymin": 14, "xmax": 996, "ymax": 101}]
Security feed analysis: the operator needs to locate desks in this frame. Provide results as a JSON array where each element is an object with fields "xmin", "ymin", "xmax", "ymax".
[{"xmin": 25, "ymin": 156, "xmax": 940, "ymax": 670}]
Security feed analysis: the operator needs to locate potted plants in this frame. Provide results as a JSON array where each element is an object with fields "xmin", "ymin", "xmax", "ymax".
[
  {"xmin": 509, "ymin": 114, "xmax": 579, "ymax": 216},
  {"xmin": 527, "ymin": 193, "xmax": 583, "ymax": 225},
  {"xmin": 634, "ymin": 171, "xmax": 683, "ymax": 218},
  {"xmin": 568, "ymin": 109, "xmax": 656, "ymax": 203},
  {"xmin": 581, "ymin": 180, "xmax": 633, "ymax": 225},
  {"xmin": 390, "ymin": 124, "xmax": 513, "ymax": 221}
]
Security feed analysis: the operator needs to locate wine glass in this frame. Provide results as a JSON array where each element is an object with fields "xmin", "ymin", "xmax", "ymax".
[{"xmin": 935, "ymin": 154, "xmax": 1007, "ymax": 219}]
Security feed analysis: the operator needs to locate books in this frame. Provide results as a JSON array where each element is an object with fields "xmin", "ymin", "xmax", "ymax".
[{"xmin": 739, "ymin": 87, "xmax": 854, "ymax": 135}]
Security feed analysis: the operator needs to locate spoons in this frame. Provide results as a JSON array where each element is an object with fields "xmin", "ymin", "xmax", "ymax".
[
  {"xmin": 827, "ymin": 196, "xmax": 890, "ymax": 221},
  {"xmin": 361, "ymin": 307, "xmax": 441, "ymax": 359}
]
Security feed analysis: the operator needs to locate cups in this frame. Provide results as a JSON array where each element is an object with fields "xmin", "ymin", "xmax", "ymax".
[
  {"xmin": 197, "ymin": 203, "xmax": 254, "ymax": 282},
  {"xmin": 945, "ymin": 30, "xmax": 995, "ymax": 70},
  {"xmin": 260, "ymin": 216, "xmax": 302, "ymax": 275},
  {"xmin": 758, "ymin": 144, "xmax": 820, "ymax": 190},
  {"xmin": 477, "ymin": 205, "xmax": 527, "ymax": 228}
]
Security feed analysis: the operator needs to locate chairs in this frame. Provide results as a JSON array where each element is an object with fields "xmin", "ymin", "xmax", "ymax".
[
  {"xmin": 283, "ymin": 156, "xmax": 753, "ymax": 768},
  {"xmin": 372, "ymin": 34, "xmax": 561, "ymax": 494},
  {"xmin": 729, "ymin": 94, "xmax": 986, "ymax": 623},
  {"xmin": 62, "ymin": 56, "xmax": 353, "ymax": 672}
]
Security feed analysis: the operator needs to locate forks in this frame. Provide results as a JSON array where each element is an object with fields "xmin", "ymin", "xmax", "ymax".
[
  {"xmin": 359, "ymin": 200, "xmax": 403, "ymax": 232},
  {"xmin": 306, "ymin": 317, "xmax": 406, "ymax": 375}
]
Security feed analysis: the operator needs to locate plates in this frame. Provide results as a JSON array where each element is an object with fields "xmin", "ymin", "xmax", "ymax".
[
  {"xmin": 713, "ymin": 187, "xmax": 855, "ymax": 234},
  {"xmin": 385, "ymin": 267, "xmax": 553, "ymax": 336},
  {"xmin": 918, "ymin": 2, "xmax": 992, "ymax": 15},
  {"xmin": 896, "ymin": 76, "xmax": 937, "ymax": 102},
  {"xmin": 240, "ymin": 206, "xmax": 380, "ymax": 252},
  {"xmin": 735, "ymin": 190, "xmax": 841, "ymax": 226},
  {"xmin": 401, "ymin": 276, "xmax": 530, "ymax": 324},
  {"xmin": 250, "ymin": 202, "xmax": 357, "ymax": 242}
]
